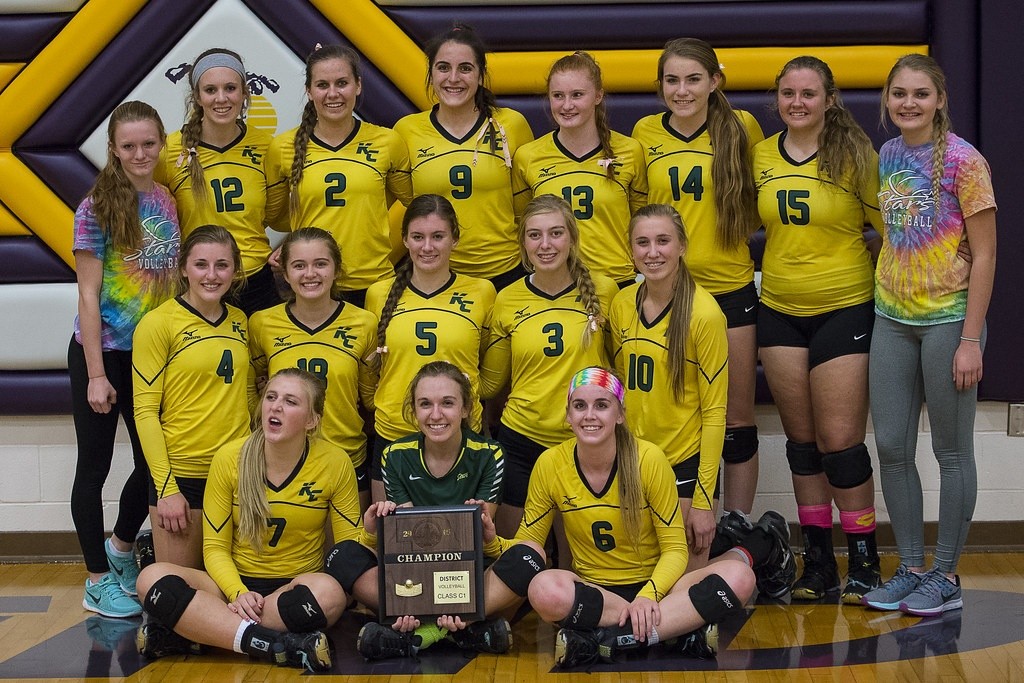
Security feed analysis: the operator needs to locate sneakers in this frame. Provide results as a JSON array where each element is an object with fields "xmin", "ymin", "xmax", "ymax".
[
  {"xmin": 554, "ymin": 627, "xmax": 615, "ymax": 669},
  {"xmin": 357, "ymin": 622, "xmax": 422, "ymax": 661},
  {"xmin": 841, "ymin": 554, "xmax": 884, "ymax": 605},
  {"xmin": 860, "ymin": 563, "xmax": 922, "ymax": 610},
  {"xmin": 717, "ymin": 509, "xmax": 768, "ymax": 593},
  {"xmin": 137, "ymin": 622, "xmax": 202, "ymax": 657},
  {"xmin": 105, "ymin": 538, "xmax": 140, "ymax": 595},
  {"xmin": 271, "ymin": 629, "xmax": 332, "ymax": 672},
  {"xmin": 446, "ymin": 618, "xmax": 513, "ymax": 653},
  {"xmin": 84, "ymin": 618, "xmax": 140, "ymax": 649},
  {"xmin": 752, "ymin": 510, "xmax": 798, "ymax": 598},
  {"xmin": 791, "ymin": 558, "xmax": 841, "ymax": 600},
  {"xmin": 82, "ymin": 572, "xmax": 144, "ymax": 617},
  {"xmin": 653, "ymin": 624, "xmax": 718, "ymax": 658},
  {"xmin": 899, "ymin": 566, "xmax": 963, "ymax": 616}
]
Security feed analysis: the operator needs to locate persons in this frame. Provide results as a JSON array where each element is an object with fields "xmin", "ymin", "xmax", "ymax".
[
  {"xmin": 136, "ymin": 26, "xmax": 766, "ymax": 579},
  {"xmin": 463, "ymin": 367, "xmax": 755, "ymax": 670},
  {"xmin": 66, "ymin": 102, "xmax": 188, "ymax": 618},
  {"xmin": 321, "ymin": 361, "xmax": 546, "ymax": 661},
  {"xmin": 859, "ymin": 52, "xmax": 999, "ymax": 617},
  {"xmin": 753, "ymin": 56, "xmax": 973, "ymax": 607},
  {"xmin": 135, "ymin": 368, "xmax": 404, "ymax": 672}
]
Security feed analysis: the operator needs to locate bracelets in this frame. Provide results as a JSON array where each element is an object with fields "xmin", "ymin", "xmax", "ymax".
[
  {"xmin": 88, "ymin": 374, "xmax": 107, "ymax": 379},
  {"xmin": 960, "ymin": 336, "xmax": 981, "ymax": 342}
]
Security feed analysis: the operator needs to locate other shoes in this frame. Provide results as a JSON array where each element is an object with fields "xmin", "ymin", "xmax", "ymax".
[{"xmin": 136, "ymin": 528, "xmax": 155, "ymax": 563}]
